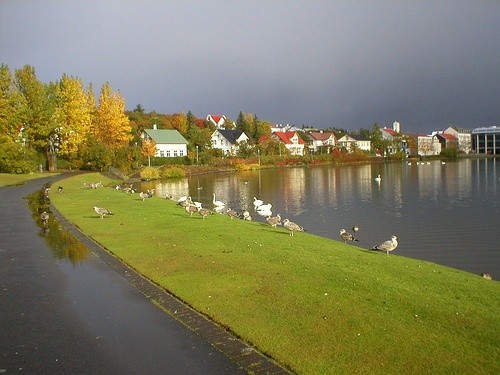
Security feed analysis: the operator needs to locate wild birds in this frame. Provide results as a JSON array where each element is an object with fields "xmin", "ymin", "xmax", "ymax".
[
  {"xmin": 481, "ymin": 271, "xmax": 493, "ymax": 280},
  {"xmin": 38, "ymin": 177, "xmax": 398, "ymax": 254}
]
